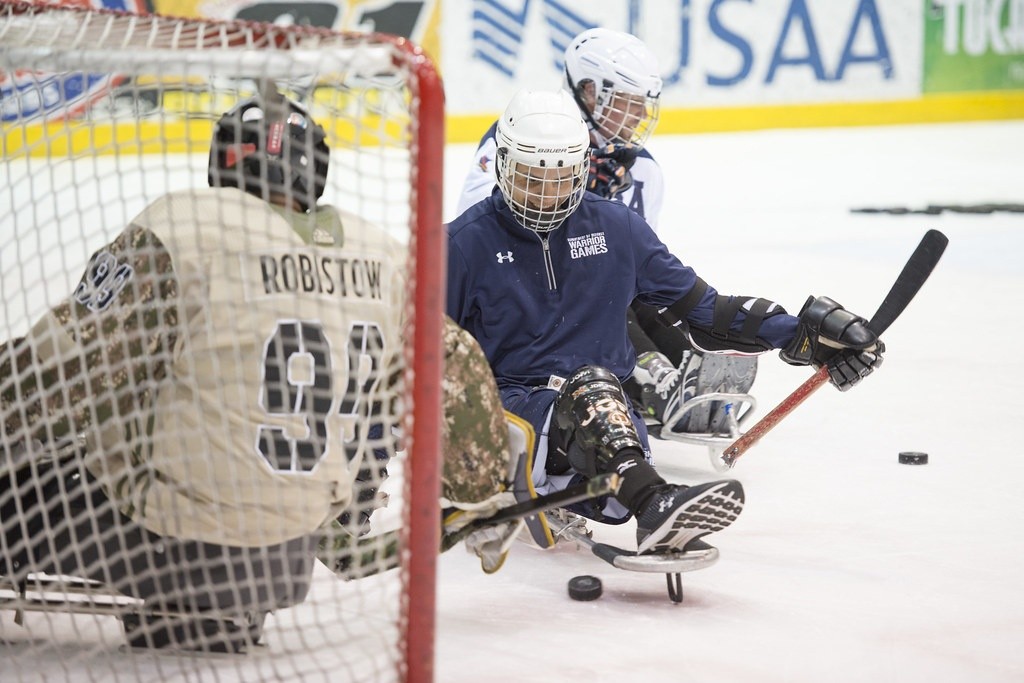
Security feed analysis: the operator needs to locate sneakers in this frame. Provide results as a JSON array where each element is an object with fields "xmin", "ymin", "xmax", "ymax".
[
  {"xmin": 635, "ymin": 479, "xmax": 745, "ymax": 556},
  {"xmin": 630, "ymin": 345, "xmax": 758, "ymax": 433}
]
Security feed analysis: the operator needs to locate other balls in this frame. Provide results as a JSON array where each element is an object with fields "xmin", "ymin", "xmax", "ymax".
[
  {"xmin": 899, "ymin": 451, "xmax": 930, "ymax": 467},
  {"xmin": 567, "ymin": 574, "xmax": 602, "ymax": 601}
]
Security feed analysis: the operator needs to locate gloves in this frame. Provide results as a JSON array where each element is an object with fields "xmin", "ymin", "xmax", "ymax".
[{"xmin": 778, "ymin": 295, "xmax": 886, "ymax": 392}]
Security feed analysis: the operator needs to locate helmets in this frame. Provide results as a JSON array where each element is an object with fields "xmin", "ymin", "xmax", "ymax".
[
  {"xmin": 495, "ymin": 83, "xmax": 590, "ymax": 233},
  {"xmin": 208, "ymin": 93, "xmax": 330, "ymax": 212},
  {"xmin": 560, "ymin": 27, "xmax": 662, "ymax": 152}
]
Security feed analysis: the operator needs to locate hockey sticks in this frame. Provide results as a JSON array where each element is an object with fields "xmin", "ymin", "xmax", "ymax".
[
  {"xmin": 316, "ymin": 469, "xmax": 623, "ymax": 582},
  {"xmin": 719, "ymin": 229, "xmax": 950, "ymax": 470}
]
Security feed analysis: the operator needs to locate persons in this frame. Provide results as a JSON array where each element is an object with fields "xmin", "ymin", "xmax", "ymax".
[
  {"xmin": 457, "ymin": 29, "xmax": 761, "ymax": 434},
  {"xmin": 0, "ymin": 87, "xmax": 527, "ymax": 655},
  {"xmin": 439, "ymin": 81, "xmax": 886, "ymax": 560}
]
